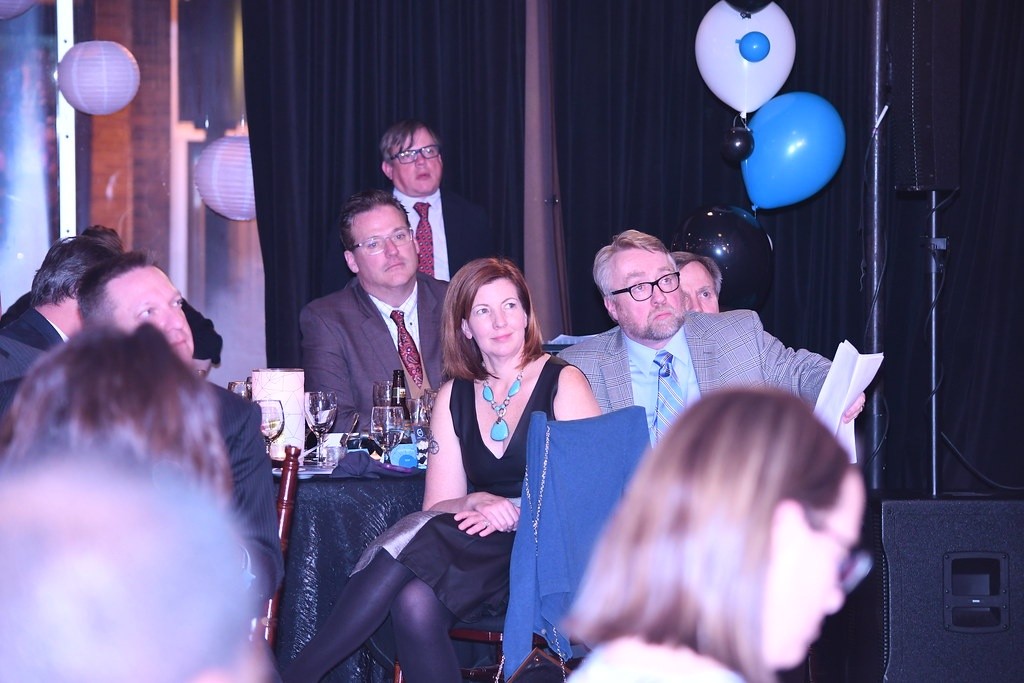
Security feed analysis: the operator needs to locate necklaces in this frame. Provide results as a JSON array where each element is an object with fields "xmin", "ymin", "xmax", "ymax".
[{"xmin": 482, "ymin": 368, "xmax": 523, "ymax": 441}]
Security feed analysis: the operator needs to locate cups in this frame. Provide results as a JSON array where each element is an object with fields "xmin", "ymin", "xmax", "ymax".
[
  {"xmin": 372, "ymin": 381, "xmax": 393, "ymax": 431},
  {"xmin": 406, "ymin": 387, "xmax": 438, "ymax": 430},
  {"xmin": 227, "ymin": 381, "xmax": 252, "ymax": 401}
]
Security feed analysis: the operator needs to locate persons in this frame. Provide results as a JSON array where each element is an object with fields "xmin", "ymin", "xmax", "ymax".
[
  {"xmin": 382, "ymin": 120, "xmax": 490, "ymax": 282},
  {"xmin": 671, "ymin": 252, "xmax": 722, "ymax": 314},
  {"xmin": 558, "ymin": 230, "xmax": 864, "ymax": 449},
  {"xmin": 83, "ymin": 255, "xmax": 286, "ymax": 607},
  {"xmin": 565, "ymin": 387, "xmax": 862, "ymax": 683},
  {"xmin": 0, "ymin": 239, "xmax": 112, "ymax": 412},
  {"xmin": 281, "ymin": 258, "xmax": 602, "ymax": 683},
  {"xmin": 300, "ymin": 196, "xmax": 450, "ymax": 435},
  {"xmin": 0, "ymin": 323, "xmax": 276, "ymax": 683}
]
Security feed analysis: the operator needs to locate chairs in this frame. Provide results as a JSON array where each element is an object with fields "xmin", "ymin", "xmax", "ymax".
[{"xmin": 395, "ymin": 406, "xmax": 650, "ymax": 683}]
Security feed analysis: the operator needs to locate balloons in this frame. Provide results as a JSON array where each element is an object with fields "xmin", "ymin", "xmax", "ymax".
[
  {"xmin": 720, "ymin": 127, "xmax": 754, "ymax": 160},
  {"xmin": 742, "ymin": 92, "xmax": 846, "ymax": 209},
  {"xmin": 695, "ymin": 2, "xmax": 795, "ymax": 118},
  {"xmin": 672, "ymin": 205, "xmax": 771, "ymax": 313},
  {"xmin": 740, "ymin": 31, "xmax": 771, "ymax": 62},
  {"xmin": 725, "ymin": 0, "xmax": 774, "ymax": 17}
]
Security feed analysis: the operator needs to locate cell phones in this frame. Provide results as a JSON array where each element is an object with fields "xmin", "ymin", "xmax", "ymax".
[{"xmin": 383, "ymin": 462, "xmax": 411, "ymax": 473}]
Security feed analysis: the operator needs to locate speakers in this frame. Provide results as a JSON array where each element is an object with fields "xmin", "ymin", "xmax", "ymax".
[
  {"xmin": 891, "ymin": 0, "xmax": 963, "ymax": 193},
  {"xmin": 836, "ymin": 490, "xmax": 1022, "ymax": 683}
]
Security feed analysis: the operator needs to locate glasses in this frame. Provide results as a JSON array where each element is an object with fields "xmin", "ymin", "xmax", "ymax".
[
  {"xmin": 609, "ymin": 271, "xmax": 681, "ymax": 302},
  {"xmin": 348, "ymin": 227, "xmax": 415, "ymax": 256},
  {"xmin": 799, "ymin": 513, "xmax": 873, "ymax": 594},
  {"xmin": 386, "ymin": 143, "xmax": 442, "ymax": 164}
]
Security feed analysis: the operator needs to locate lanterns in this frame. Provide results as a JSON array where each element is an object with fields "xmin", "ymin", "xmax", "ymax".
[
  {"xmin": 59, "ymin": 40, "xmax": 139, "ymax": 116},
  {"xmin": 191, "ymin": 135, "xmax": 259, "ymax": 221}
]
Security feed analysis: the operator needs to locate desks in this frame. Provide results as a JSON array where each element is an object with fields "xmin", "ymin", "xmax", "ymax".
[{"xmin": 273, "ymin": 471, "xmax": 426, "ymax": 683}]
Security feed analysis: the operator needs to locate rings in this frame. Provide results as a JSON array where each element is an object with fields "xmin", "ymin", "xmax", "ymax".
[{"xmin": 483, "ymin": 519, "xmax": 489, "ymax": 527}]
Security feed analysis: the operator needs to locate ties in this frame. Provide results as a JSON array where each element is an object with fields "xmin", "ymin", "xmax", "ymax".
[
  {"xmin": 413, "ymin": 202, "xmax": 436, "ymax": 278},
  {"xmin": 390, "ymin": 310, "xmax": 423, "ymax": 390},
  {"xmin": 650, "ymin": 349, "xmax": 685, "ymax": 445}
]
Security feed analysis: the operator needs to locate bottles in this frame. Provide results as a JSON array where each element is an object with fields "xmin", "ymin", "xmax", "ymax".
[{"xmin": 390, "ymin": 369, "xmax": 414, "ymax": 444}]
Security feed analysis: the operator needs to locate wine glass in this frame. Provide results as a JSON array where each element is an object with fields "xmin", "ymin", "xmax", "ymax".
[
  {"xmin": 370, "ymin": 406, "xmax": 405, "ymax": 464},
  {"xmin": 253, "ymin": 400, "xmax": 285, "ymax": 456},
  {"xmin": 304, "ymin": 392, "xmax": 338, "ymax": 470}
]
